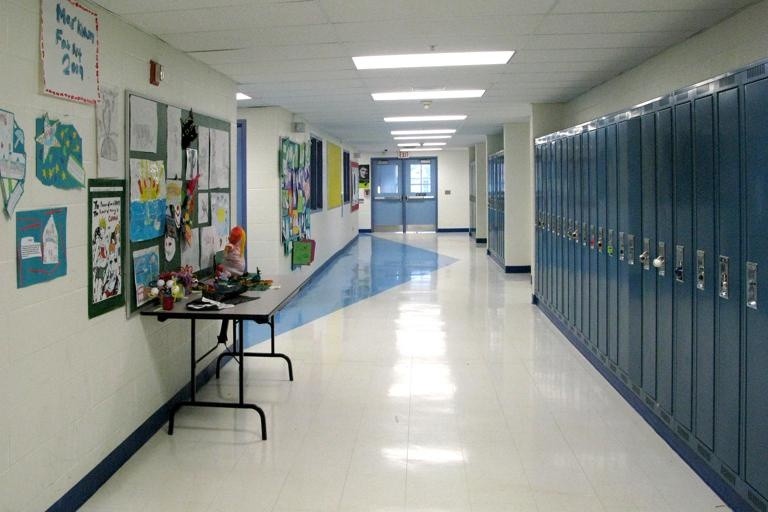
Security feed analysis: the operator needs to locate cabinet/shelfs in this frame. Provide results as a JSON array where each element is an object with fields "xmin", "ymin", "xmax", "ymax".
[
  {"xmin": 692, "ymin": 80, "xmax": 714, "ymax": 489},
  {"xmin": 654, "ymin": 96, "xmax": 668, "ymax": 439},
  {"xmin": 625, "ymin": 106, "xmax": 637, "ymax": 409},
  {"xmin": 639, "ymin": 98, "xmax": 654, "ymax": 418},
  {"xmin": 715, "ymin": 76, "xmax": 740, "ymax": 510},
  {"xmin": 669, "ymin": 86, "xmax": 690, "ymax": 460},
  {"xmin": 533, "ymin": 110, "xmax": 620, "ymax": 396},
  {"xmin": 741, "ymin": 59, "xmax": 767, "ymax": 512},
  {"xmin": 488, "ymin": 151, "xmax": 506, "ymax": 271}
]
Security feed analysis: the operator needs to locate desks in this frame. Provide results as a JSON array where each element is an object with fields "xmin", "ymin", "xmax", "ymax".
[{"xmin": 142, "ymin": 274, "xmax": 307, "ymax": 440}]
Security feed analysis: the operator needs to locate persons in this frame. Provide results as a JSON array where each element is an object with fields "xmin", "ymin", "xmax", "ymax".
[{"xmin": 360, "ymin": 167, "xmax": 368, "ymax": 179}]
